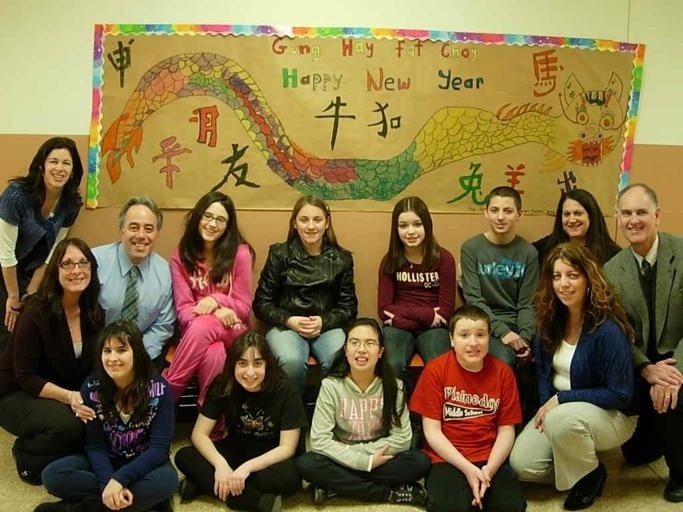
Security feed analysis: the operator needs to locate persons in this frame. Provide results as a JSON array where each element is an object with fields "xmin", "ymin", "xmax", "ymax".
[
  {"xmin": 510, "ymin": 243, "xmax": 649, "ymax": 510},
  {"xmin": 1, "ymin": 139, "xmax": 84, "ymax": 344},
  {"xmin": 2, "ymin": 238, "xmax": 104, "ymax": 485},
  {"xmin": 600, "ymin": 182, "xmax": 683, "ymax": 502},
  {"xmin": 89, "ymin": 192, "xmax": 259, "ymax": 440},
  {"xmin": 252, "ymin": 194, "xmax": 358, "ymax": 390},
  {"xmin": 378, "ymin": 186, "xmax": 623, "ymax": 452},
  {"xmin": 31, "ymin": 304, "xmax": 526, "ymax": 511}
]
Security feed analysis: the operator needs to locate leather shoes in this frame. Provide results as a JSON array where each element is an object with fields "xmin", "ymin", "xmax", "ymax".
[
  {"xmin": 664, "ymin": 474, "xmax": 683, "ymax": 501},
  {"xmin": 12, "ymin": 438, "xmax": 41, "ymax": 484}
]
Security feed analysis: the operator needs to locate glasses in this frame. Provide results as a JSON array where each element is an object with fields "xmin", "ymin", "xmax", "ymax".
[
  {"xmin": 350, "ymin": 341, "xmax": 378, "ymax": 347},
  {"xmin": 59, "ymin": 261, "xmax": 91, "ymax": 268},
  {"xmin": 203, "ymin": 213, "xmax": 227, "ymax": 224}
]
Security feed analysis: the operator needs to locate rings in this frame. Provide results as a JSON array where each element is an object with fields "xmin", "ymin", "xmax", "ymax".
[{"xmin": 665, "ymin": 396, "xmax": 671, "ymax": 398}]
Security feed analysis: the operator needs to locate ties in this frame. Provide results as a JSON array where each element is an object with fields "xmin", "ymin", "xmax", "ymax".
[{"xmin": 121, "ymin": 267, "xmax": 138, "ymax": 324}]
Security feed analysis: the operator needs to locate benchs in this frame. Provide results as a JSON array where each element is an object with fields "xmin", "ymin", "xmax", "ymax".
[{"xmin": 164, "ymin": 345, "xmax": 425, "ymax": 408}]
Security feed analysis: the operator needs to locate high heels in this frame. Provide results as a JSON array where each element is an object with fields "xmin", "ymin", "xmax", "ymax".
[{"xmin": 564, "ymin": 463, "xmax": 607, "ymax": 509}]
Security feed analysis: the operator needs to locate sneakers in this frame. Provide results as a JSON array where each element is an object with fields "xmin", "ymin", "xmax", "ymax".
[
  {"xmin": 260, "ymin": 493, "xmax": 282, "ymax": 512},
  {"xmin": 178, "ymin": 478, "xmax": 205, "ymax": 499},
  {"xmin": 313, "ymin": 486, "xmax": 338, "ymax": 505},
  {"xmin": 388, "ymin": 481, "xmax": 427, "ymax": 506}
]
